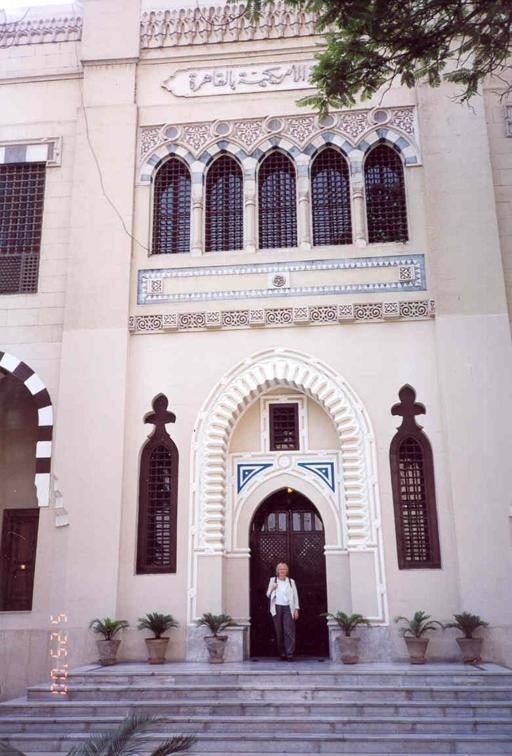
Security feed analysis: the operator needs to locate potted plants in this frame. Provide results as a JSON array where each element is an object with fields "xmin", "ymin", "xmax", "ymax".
[
  {"xmin": 323, "ymin": 609, "xmax": 490, "ymax": 664},
  {"xmin": 89, "ymin": 611, "xmax": 240, "ymax": 666}
]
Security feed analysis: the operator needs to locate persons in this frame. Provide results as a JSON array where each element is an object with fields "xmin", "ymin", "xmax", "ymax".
[{"xmin": 266, "ymin": 561, "xmax": 301, "ymax": 662}]
